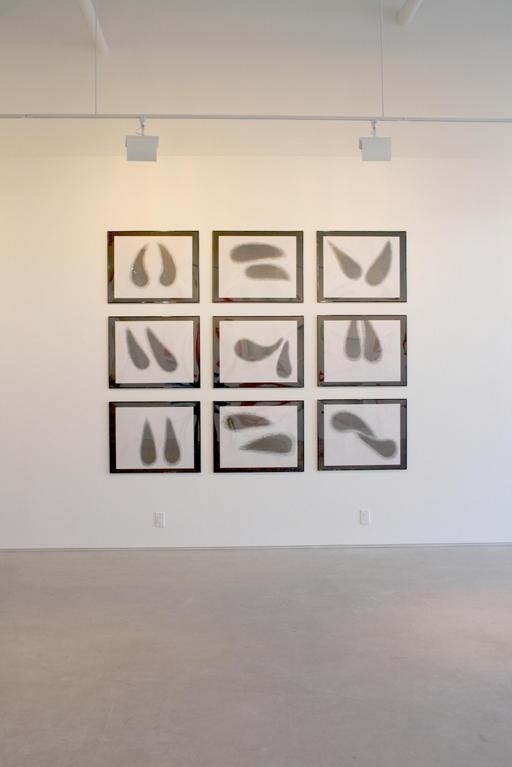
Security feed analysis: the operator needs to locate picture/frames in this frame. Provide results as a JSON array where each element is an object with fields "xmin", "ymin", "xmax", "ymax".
[
  {"xmin": 212, "ymin": 315, "xmax": 304, "ymax": 387},
  {"xmin": 317, "ymin": 314, "xmax": 406, "ymax": 386},
  {"xmin": 108, "ymin": 231, "xmax": 201, "ymax": 305},
  {"xmin": 316, "ymin": 399, "xmax": 407, "ymax": 472},
  {"xmin": 212, "ymin": 231, "xmax": 304, "ymax": 303},
  {"xmin": 110, "ymin": 401, "xmax": 202, "ymax": 473},
  {"xmin": 212, "ymin": 400, "xmax": 304, "ymax": 472},
  {"xmin": 316, "ymin": 232, "xmax": 406, "ymax": 303},
  {"xmin": 110, "ymin": 316, "xmax": 202, "ymax": 390}
]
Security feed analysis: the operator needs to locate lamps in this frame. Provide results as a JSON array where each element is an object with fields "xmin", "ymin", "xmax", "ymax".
[
  {"xmin": 125, "ymin": 116, "xmax": 161, "ymax": 162},
  {"xmin": 360, "ymin": 121, "xmax": 393, "ymax": 163}
]
